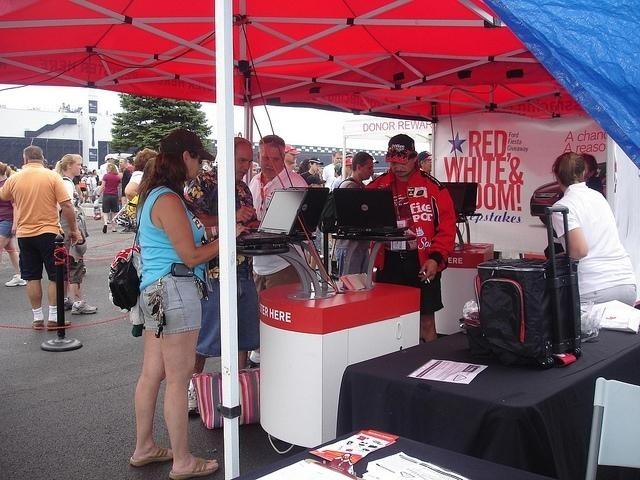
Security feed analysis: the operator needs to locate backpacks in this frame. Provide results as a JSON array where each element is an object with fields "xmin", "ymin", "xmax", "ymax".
[{"xmin": 108, "ymin": 256, "xmax": 141, "ymax": 314}]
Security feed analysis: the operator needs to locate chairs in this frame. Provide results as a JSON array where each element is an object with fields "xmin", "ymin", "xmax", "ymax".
[{"xmin": 585, "ymin": 377, "xmax": 640, "ymax": 480}]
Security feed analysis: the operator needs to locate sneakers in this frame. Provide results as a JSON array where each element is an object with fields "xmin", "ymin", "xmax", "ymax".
[
  {"xmin": 31, "ymin": 319, "xmax": 44, "ymax": 330},
  {"xmin": 187, "ymin": 388, "xmax": 198, "ymax": 417},
  {"xmin": 47, "ymin": 320, "xmax": 72, "ymax": 330},
  {"xmin": 102, "ymin": 225, "xmax": 118, "ymax": 234},
  {"xmin": 70, "ymin": 300, "xmax": 98, "ymax": 315},
  {"xmin": 4, "ymin": 273, "xmax": 27, "ymax": 287},
  {"xmin": 64, "ymin": 297, "xmax": 74, "ymax": 311}
]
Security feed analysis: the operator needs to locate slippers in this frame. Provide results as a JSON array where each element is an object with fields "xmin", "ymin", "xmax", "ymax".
[
  {"xmin": 129, "ymin": 446, "xmax": 173, "ymax": 467},
  {"xmin": 168, "ymin": 457, "xmax": 219, "ymax": 480}
]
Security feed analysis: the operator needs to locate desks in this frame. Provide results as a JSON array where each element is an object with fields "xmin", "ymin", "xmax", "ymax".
[
  {"xmin": 231, "ymin": 429, "xmax": 549, "ymax": 479},
  {"xmin": 336, "ymin": 329, "xmax": 640, "ymax": 479}
]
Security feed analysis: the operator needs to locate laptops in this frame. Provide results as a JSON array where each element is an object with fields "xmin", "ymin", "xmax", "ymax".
[
  {"xmin": 286, "ymin": 187, "xmax": 330, "ymax": 234},
  {"xmin": 333, "ymin": 188, "xmax": 406, "ymax": 236},
  {"xmin": 237, "ymin": 189, "xmax": 308, "ymax": 244},
  {"xmin": 443, "ymin": 182, "xmax": 477, "ymax": 212}
]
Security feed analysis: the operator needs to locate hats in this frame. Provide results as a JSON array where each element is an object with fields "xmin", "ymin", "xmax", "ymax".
[
  {"xmin": 159, "ymin": 128, "xmax": 216, "ymax": 162},
  {"xmin": 308, "ymin": 157, "xmax": 324, "ymax": 165},
  {"xmin": 284, "ymin": 144, "xmax": 301, "ymax": 155},
  {"xmin": 417, "ymin": 150, "xmax": 432, "ymax": 163},
  {"xmin": 384, "ymin": 133, "xmax": 418, "ymax": 165}
]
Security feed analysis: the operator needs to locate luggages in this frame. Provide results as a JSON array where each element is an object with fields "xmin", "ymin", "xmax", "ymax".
[{"xmin": 474, "ymin": 205, "xmax": 584, "ymax": 370}]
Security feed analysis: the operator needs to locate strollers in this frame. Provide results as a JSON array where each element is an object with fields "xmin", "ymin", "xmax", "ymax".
[{"xmin": 110, "ymin": 199, "xmax": 132, "ymax": 235}]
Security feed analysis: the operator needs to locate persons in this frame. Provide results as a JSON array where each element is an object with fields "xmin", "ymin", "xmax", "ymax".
[
  {"xmin": 185, "ymin": 137, "xmax": 263, "ymax": 415},
  {"xmin": 248, "ymin": 134, "xmax": 309, "ymax": 298},
  {"xmin": 365, "ymin": 134, "xmax": 456, "ymax": 341},
  {"xmin": 417, "ymin": 151, "xmax": 431, "ymax": 175},
  {"xmin": 550, "ymin": 151, "xmax": 637, "ymax": 307},
  {"xmin": 203, "ymin": 144, "xmax": 379, "ymax": 283},
  {"xmin": 581, "ymin": 153, "xmax": 605, "ymax": 197},
  {"xmin": 0, "ymin": 144, "xmax": 159, "ymax": 328},
  {"xmin": 130, "ymin": 129, "xmax": 252, "ymax": 480}
]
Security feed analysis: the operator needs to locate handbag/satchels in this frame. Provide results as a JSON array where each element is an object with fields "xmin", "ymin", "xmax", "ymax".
[{"xmin": 190, "ymin": 367, "xmax": 260, "ymax": 431}]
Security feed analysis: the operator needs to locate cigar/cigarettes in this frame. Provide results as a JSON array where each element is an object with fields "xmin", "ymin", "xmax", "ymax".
[{"xmin": 423, "ymin": 271, "xmax": 430, "ymax": 284}]
